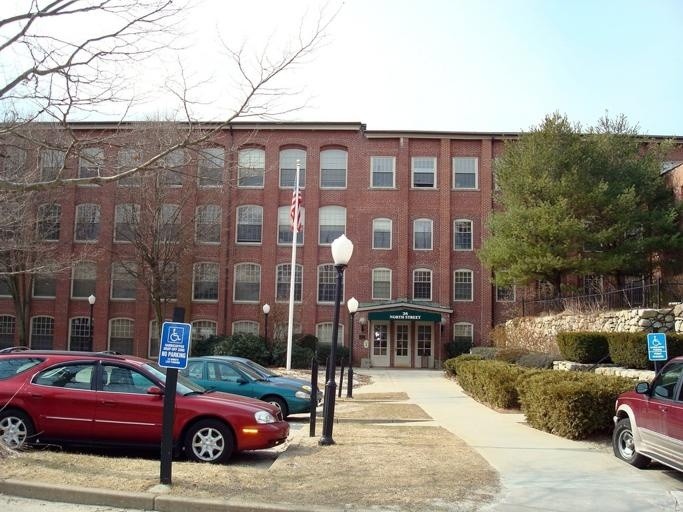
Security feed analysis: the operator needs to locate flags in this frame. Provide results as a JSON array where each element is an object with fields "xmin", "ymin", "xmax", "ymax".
[{"xmin": 289, "ymin": 170, "xmax": 302, "ymax": 232}]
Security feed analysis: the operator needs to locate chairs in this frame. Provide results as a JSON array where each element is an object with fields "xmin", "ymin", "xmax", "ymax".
[
  {"xmin": 102, "ymin": 371, "xmax": 113, "ymax": 391},
  {"xmin": 108, "ymin": 369, "xmax": 130, "ymax": 393}
]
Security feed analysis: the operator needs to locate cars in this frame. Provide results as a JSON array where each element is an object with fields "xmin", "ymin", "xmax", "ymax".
[{"xmin": 612, "ymin": 356, "xmax": 683, "ymax": 472}]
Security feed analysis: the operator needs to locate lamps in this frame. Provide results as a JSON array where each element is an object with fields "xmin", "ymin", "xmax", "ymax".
[
  {"xmin": 359, "ymin": 318, "xmax": 367, "ymax": 331},
  {"xmin": 441, "ymin": 318, "xmax": 447, "ymax": 333}
]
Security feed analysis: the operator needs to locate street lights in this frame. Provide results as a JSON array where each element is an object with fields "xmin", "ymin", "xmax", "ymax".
[
  {"xmin": 347, "ymin": 296, "xmax": 359, "ymax": 397},
  {"xmin": 263, "ymin": 303, "xmax": 270, "ymax": 346},
  {"xmin": 318, "ymin": 234, "xmax": 355, "ymax": 445},
  {"xmin": 88, "ymin": 294, "xmax": 96, "ymax": 351}
]
